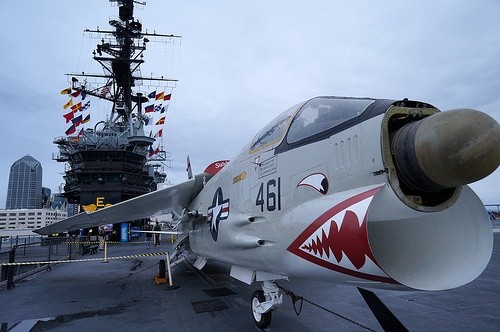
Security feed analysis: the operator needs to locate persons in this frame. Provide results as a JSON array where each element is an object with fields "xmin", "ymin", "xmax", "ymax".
[
  {"xmin": 98, "ymin": 224, "xmax": 107, "ymax": 250},
  {"xmin": 160, "ymin": 222, "xmax": 173, "ymax": 229},
  {"xmin": 145, "ymin": 224, "xmax": 153, "ymax": 249},
  {"xmin": 154, "ymin": 221, "xmax": 161, "ymax": 247},
  {"xmin": 491, "ymin": 211, "xmax": 495, "ymax": 220}
]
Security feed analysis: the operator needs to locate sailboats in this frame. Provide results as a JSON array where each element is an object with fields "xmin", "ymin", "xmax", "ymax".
[{"xmin": 51, "ymin": 0, "xmax": 183, "ymax": 227}]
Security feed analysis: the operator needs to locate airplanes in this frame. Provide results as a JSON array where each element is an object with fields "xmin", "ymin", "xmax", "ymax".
[{"xmin": 32, "ymin": 95, "xmax": 500, "ymax": 329}]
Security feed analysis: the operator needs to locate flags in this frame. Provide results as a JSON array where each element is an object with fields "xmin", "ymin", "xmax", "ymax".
[
  {"xmin": 60, "ymin": 85, "xmax": 90, "ymax": 142},
  {"xmin": 145, "ymin": 91, "xmax": 172, "ymax": 159},
  {"xmin": 99, "ymin": 82, "xmax": 112, "ymax": 98}
]
